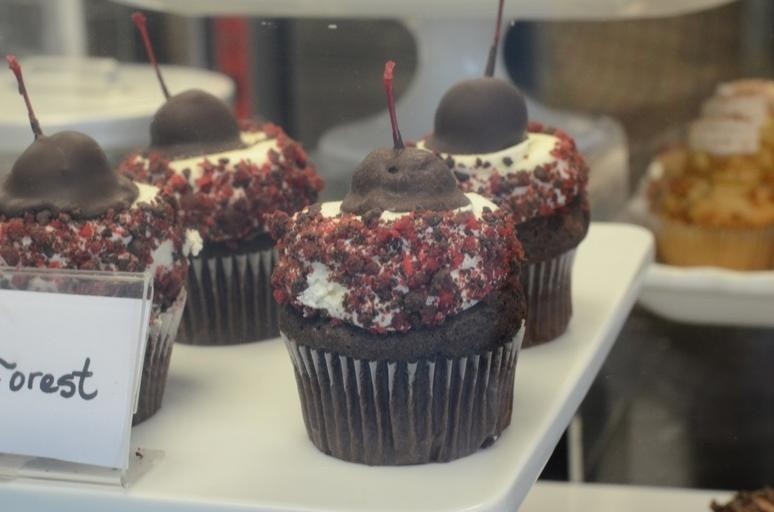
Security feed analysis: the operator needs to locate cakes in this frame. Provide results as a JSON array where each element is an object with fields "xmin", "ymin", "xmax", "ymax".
[
  {"xmin": 405, "ymin": 0, "xmax": 592, "ymax": 349},
  {"xmin": 0, "ymin": 54, "xmax": 187, "ymax": 428},
  {"xmin": 117, "ymin": 9, "xmax": 325, "ymax": 347},
  {"xmin": 262, "ymin": 61, "xmax": 529, "ymax": 467}
]
